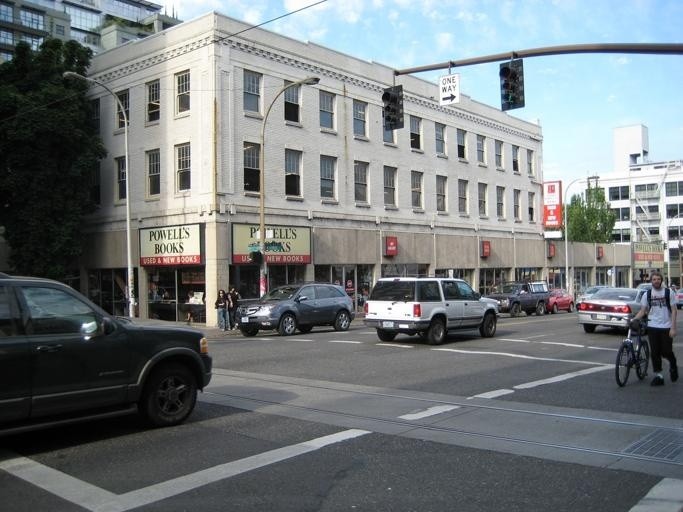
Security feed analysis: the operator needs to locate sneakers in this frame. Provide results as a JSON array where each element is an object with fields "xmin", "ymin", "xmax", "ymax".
[
  {"xmin": 221, "ymin": 324, "xmax": 238, "ymax": 332},
  {"xmin": 650, "ymin": 375, "xmax": 665, "ymax": 387},
  {"xmin": 670, "ymin": 365, "xmax": 679, "ymax": 383}
]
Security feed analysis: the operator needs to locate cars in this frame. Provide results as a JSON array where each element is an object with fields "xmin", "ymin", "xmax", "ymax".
[
  {"xmin": 674, "ymin": 288, "xmax": 682, "ymax": 309},
  {"xmin": 544, "ymin": 289, "xmax": 573, "ymax": 314},
  {"xmin": 234, "ymin": 281, "xmax": 354, "ymax": 337},
  {"xmin": 0, "ymin": 271, "xmax": 211, "ymax": 438},
  {"xmin": 634, "ymin": 282, "xmax": 667, "ymax": 291},
  {"xmin": 575, "ymin": 286, "xmax": 614, "ymax": 310},
  {"xmin": 576, "ymin": 287, "xmax": 647, "ymax": 336},
  {"xmin": 361, "ymin": 278, "xmax": 498, "ymax": 345}
]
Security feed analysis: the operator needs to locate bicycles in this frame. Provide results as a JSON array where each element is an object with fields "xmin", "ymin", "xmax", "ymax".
[{"xmin": 614, "ymin": 317, "xmax": 649, "ymax": 387}]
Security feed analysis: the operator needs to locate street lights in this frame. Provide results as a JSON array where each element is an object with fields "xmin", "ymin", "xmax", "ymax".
[
  {"xmin": 563, "ymin": 178, "xmax": 586, "ymax": 292},
  {"xmin": 61, "ymin": 68, "xmax": 136, "ymax": 319},
  {"xmin": 258, "ymin": 77, "xmax": 319, "ymax": 299}
]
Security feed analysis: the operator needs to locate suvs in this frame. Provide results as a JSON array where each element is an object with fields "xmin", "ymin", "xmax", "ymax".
[{"xmin": 481, "ymin": 279, "xmax": 549, "ymax": 318}]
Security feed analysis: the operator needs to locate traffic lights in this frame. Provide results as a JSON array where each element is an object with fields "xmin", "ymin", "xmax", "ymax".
[
  {"xmin": 381, "ymin": 84, "xmax": 403, "ymax": 131},
  {"xmin": 663, "ymin": 242, "xmax": 666, "ymax": 249},
  {"xmin": 499, "ymin": 59, "xmax": 523, "ymax": 111}
]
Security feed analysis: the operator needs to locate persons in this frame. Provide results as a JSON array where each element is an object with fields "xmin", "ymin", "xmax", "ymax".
[
  {"xmin": 184, "ymin": 291, "xmax": 195, "ymax": 320},
  {"xmin": 229, "ymin": 288, "xmax": 235, "ymax": 293},
  {"xmin": 631, "ymin": 272, "xmax": 678, "ymax": 386},
  {"xmin": 162, "ymin": 288, "xmax": 169, "ymax": 298},
  {"xmin": 216, "ymin": 289, "xmax": 229, "ymax": 332},
  {"xmin": 227, "ymin": 288, "xmax": 240, "ymax": 330}
]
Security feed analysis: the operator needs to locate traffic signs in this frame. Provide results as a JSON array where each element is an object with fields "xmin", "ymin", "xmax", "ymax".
[{"xmin": 438, "ymin": 73, "xmax": 459, "ymax": 106}]
